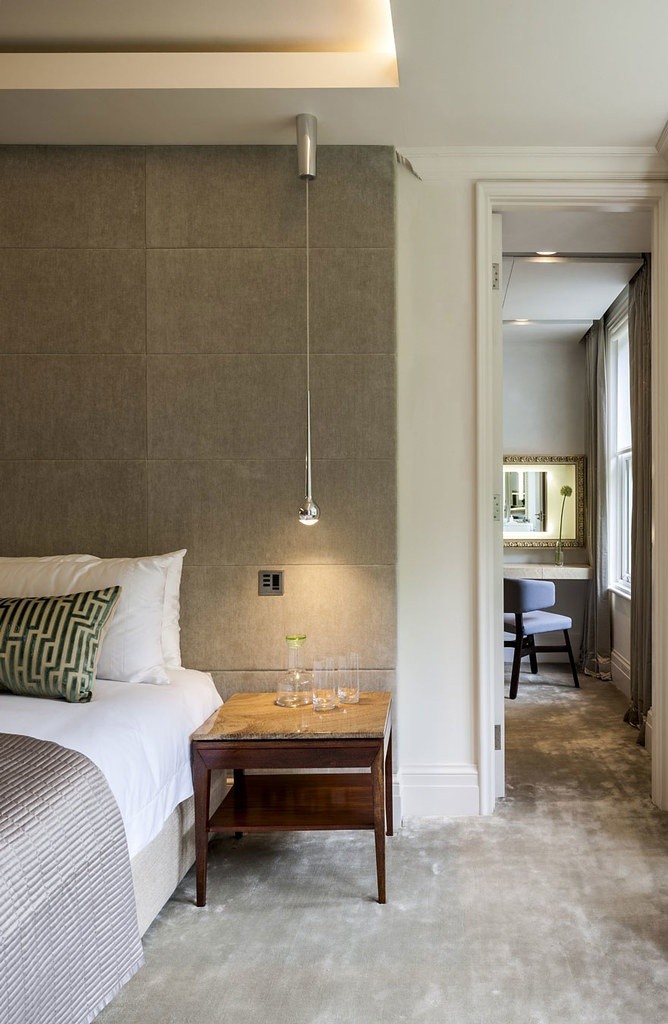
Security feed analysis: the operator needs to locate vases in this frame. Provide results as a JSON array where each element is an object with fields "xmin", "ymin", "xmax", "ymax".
[{"xmin": 555, "ymin": 541, "xmax": 564, "ymax": 568}]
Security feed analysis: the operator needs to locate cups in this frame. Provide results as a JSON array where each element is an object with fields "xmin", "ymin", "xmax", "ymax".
[
  {"xmin": 313, "ymin": 657, "xmax": 336, "ymax": 711},
  {"xmin": 337, "ymin": 653, "xmax": 360, "ymax": 704}
]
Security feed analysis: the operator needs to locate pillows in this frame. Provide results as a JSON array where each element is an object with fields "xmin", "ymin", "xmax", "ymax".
[
  {"xmin": 0, "ymin": 586, "xmax": 122, "ymax": 703},
  {"xmin": 0, "ymin": 548, "xmax": 187, "ymax": 670},
  {"xmin": 0, "ymin": 556, "xmax": 172, "ymax": 686}
]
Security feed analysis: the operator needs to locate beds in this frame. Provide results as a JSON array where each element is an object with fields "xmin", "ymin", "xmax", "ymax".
[{"xmin": 0, "ymin": 667, "xmax": 227, "ymax": 1023}]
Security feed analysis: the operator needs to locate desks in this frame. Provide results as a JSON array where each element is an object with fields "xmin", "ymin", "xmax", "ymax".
[{"xmin": 504, "ymin": 562, "xmax": 593, "ymax": 579}]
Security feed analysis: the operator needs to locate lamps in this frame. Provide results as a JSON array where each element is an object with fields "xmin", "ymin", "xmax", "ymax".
[{"xmin": 298, "ymin": 112, "xmax": 320, "ymax": 527}]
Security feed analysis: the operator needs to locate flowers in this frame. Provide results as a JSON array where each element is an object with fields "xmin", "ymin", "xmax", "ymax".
[{"xmin": 555, "ymin": 486, "xmax": 572, "ymax": 561}]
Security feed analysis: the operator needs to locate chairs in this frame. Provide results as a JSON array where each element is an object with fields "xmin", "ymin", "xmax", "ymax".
[{"xmin": 504, "ymin": 577, "xmax": 580, "ymax": 699}]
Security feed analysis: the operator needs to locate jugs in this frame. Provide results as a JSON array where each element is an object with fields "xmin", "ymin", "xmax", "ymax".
[{"xmin": 275, "ymin": 635, "xmax": 313, "ymax": 707}]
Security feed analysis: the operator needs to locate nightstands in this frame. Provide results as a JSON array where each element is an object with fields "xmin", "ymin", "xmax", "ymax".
[{"xmin": 190, "ymin": 692, "xmax": 393, "ymax": 907}]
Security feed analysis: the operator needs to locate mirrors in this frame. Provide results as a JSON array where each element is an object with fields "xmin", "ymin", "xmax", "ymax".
[
  {"xmin": 503, "ymin": 455, "xmax": 585, "ymax": 548},
  {"xmin": 507, "ymin": 472, "xmax": 528, "ymax": 523}
]
